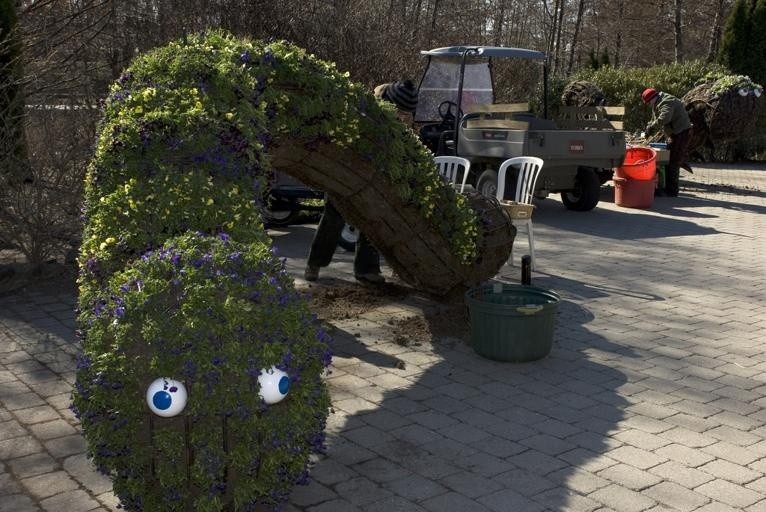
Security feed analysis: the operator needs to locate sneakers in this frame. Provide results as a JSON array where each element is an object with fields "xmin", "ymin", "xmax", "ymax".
[
  {"xmin": 354, "ymin": 271, "xmax": 386, "ymax": 284},
  {"xmin": 303, "ymin": 260, "xmax": 321, "ymax": 282}
]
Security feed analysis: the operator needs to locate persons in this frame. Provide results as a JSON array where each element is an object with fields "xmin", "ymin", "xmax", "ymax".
[
  {"xmin": 304, "ymin": 78, "xmax": 420, "ymax": 287},
  {"xmin": 640, "ymin": 88, "xmax": 693, "ymax": 197}
]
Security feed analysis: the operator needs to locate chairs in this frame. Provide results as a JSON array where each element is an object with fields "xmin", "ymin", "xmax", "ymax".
[{"xmin": 433, "ymin": 155, "xmax": 544, "ymax": 279}]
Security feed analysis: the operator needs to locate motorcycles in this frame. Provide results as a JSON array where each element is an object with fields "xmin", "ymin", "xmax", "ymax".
[{"xmin": 410, "ymin": 44, "xmax": 627, "ymax": 215}]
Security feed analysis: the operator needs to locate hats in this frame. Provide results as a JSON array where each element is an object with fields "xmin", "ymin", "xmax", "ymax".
[
  {"xmin": 380, "ymin": 76, "xmax": 419, "ymax": 114},
  {"xmin": 641, "ymin": 88, "xmax": 658, "ymax": 104}
]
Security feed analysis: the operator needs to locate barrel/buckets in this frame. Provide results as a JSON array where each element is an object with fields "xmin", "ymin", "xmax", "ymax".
[
  {"xmin": 612, "ymin": 177, "xmax": 657, "ymax": 207},
  {"xmin": 615, "ymin": 148, "xmax": 657, "ymax": 180}
]
